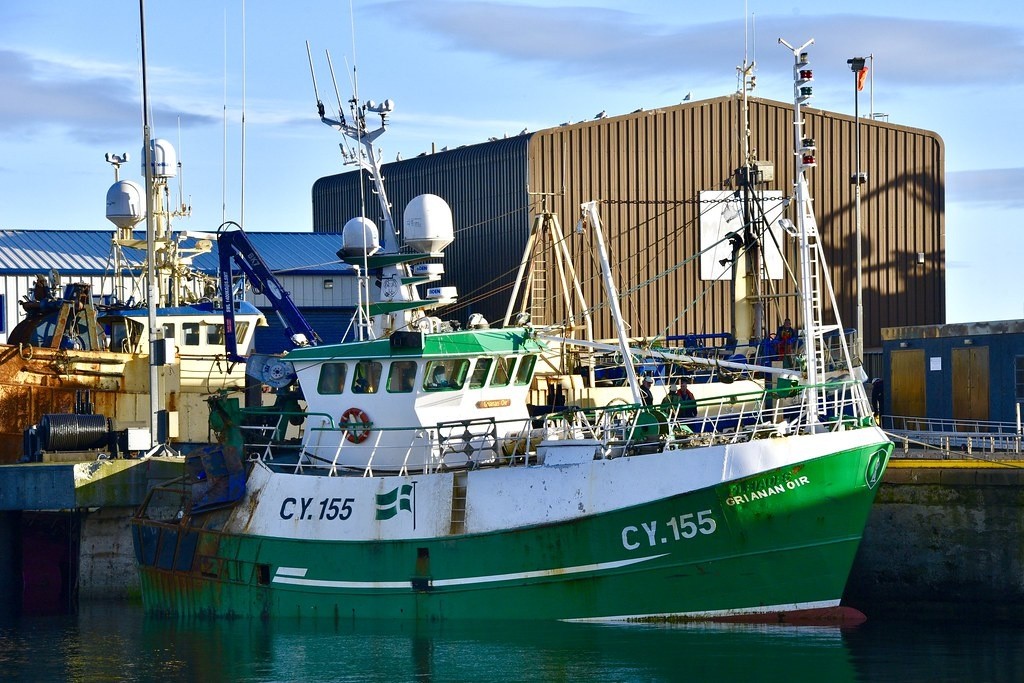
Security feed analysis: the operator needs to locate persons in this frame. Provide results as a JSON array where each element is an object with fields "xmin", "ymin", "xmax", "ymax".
[
  {"xmin": 640, "ymin": 377, "xmax": 698, "ymax": 418},
  {"xmin": 775, "ymin": 319, "xmax": 795, "ymax": 360}
]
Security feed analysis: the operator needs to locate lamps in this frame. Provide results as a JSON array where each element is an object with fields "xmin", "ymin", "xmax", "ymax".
[
  {"xmin": 778, "ymin": 219, "xmax": 801, "ymax": 237},
  {"xmin": 719, "ymin": 258, "xmax": 733, "ymax": 267},
  {"xmin": 900, "ymin": 342, "xmax": 909, "ymax": 347},
  {"xmin": 324, "ymin": 279, "xmax": 333, "ymax": 289},
  {"xmin": 290, "ymin": 333, "xmax": 310, "ymax": 348},
  {"xmin": 964, "ymin": 339, "xmax": 973, "ymax": 344}
]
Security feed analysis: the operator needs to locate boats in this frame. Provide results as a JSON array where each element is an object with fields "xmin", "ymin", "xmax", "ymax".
[{"xmin": 133, "ymin": 0, "xmax": 899, "ymax": 632}]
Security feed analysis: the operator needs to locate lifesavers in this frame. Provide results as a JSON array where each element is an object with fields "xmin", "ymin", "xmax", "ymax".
[{"xmin": 340, "ymin": 408, "xmax": 370, "ymax": 444}]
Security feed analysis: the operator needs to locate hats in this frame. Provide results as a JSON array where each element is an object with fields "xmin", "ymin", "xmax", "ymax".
[
  {"xmin": 669, "ymin": 385, "xmax": 678, "ymax": 391},
  {"xmin": 784, "ymin": 319, "xmax": 792, "ymax": 325},
  {"xmin": 645, "ymin": 376, "xmax": 653, "ymax": 383}
]
